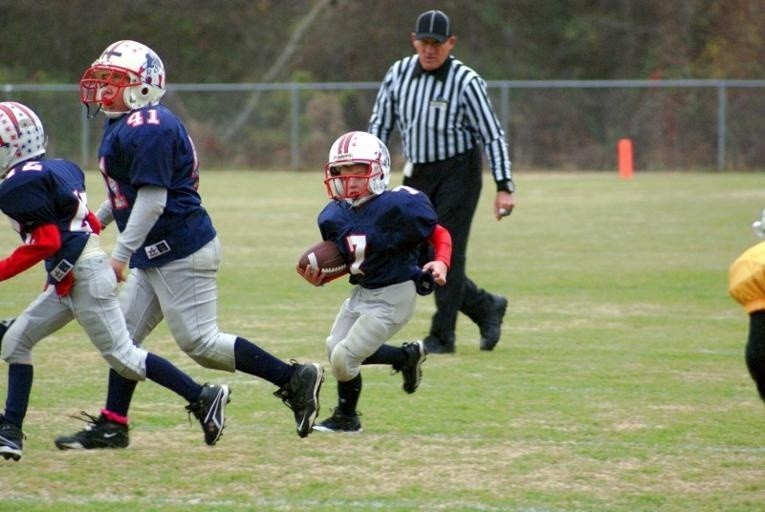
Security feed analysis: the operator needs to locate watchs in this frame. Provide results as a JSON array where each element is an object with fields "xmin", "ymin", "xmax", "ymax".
[{"xmin": 496, "ymin": 180, "xmax": 514, "ymax": 194}]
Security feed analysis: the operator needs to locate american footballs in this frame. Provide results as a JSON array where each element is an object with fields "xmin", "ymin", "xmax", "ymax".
[{"xmin": 298, "ymin": 242, "xmax": 346, "ymax": 274}]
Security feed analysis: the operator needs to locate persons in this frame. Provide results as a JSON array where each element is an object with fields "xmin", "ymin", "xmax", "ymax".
[
  {"xmin": 295, "ymin": 131, "xmax": 453, "ymax": 433},
  {"xmin": 366, "ymin": 10, "xmax": 515, "ymax": 353},
  {"xmin": 728, "ymin": 208, "xmax": 765, "ymax": 402},
  {"xmin": 0, "ymin": 101, "xmax": 232, "ymax": 462},
  {"xmin": 54, "ymin": 39, "xmax": 324, "ymax": 450}
]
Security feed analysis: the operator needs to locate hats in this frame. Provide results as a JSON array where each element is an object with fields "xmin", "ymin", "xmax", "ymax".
[{"xmin": 415, "ymin": 10, "xmax": 451, "ymax": 43}]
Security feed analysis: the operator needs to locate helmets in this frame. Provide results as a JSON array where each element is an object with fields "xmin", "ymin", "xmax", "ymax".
[
  {"xmin": 324, "ymin": 131, "xmax": 391, "ymax": 204},
  {"xmin": 0, "ymin": 101, "xmax": 46, "ymax": 179},
  {"xmin": 79, "ymin": 40, "xmax": 166, "ymax": 119}
]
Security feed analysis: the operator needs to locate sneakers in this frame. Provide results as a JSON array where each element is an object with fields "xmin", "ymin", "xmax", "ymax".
[
  {"xmin": 0, "ymin": 413, "xmax": 23, "ymax": 461},
  {"xmin": 185, "ymin": 382, "xmax": 232, "ymax": 445},
  {"xmin": 312, "ymin": 406, "xmax": 363, "ymax": 433},
  {"xmin": 55, "ymin": 413, "xmax": 128, "ymax": 449},
  {"xmin": 393, "ymin": 340, "xmax": 426, "ymax": 393},
  {"xmin": 424, "ymin": 334, "xmax": 455, "ymax": 353},
  {"xmin": 478, "ymin": 295, "xmax": 507, "ymax": 350},
  {"xmin": 273, "ymin": 358, "xmax": 325, "ymax": 437}
]
